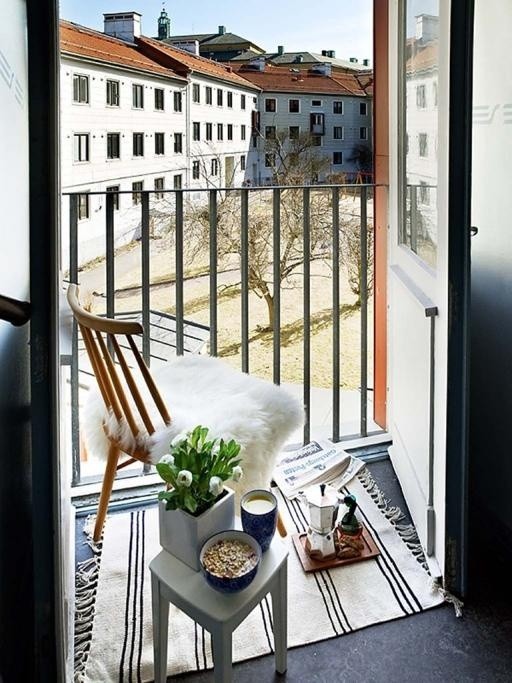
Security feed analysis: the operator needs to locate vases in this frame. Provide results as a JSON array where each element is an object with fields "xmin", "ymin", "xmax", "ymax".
[{"xmin": 155, "ymin": 484, "xmax": 234, "ymax": 571}]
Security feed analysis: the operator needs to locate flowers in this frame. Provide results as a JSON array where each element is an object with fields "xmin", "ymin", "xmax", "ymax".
[{"xmin": 155, "ymin": 427, "xmax": 245, "ymax": 515}]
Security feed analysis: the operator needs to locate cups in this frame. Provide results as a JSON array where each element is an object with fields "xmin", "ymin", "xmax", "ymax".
[{"xmin": 239, "ymin": 490, "xmax": 277, "ymax": 556}]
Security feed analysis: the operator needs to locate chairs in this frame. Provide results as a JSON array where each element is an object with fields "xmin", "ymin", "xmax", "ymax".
[{"xmin": 65, "ymin": 282, "xmax": 301, "ymax": 540}]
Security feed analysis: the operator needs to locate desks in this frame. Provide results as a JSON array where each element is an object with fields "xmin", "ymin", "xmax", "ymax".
[{"xmin": 147, "ymin": 514, "xmax": 289, "ymax": 683}]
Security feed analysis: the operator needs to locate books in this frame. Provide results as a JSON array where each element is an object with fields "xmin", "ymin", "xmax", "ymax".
[
  {"xmin": 272, "ymin": 436, "xmax": 351, "ymax": 501},
  {"xmin": 323, "ymin": 455, "xmax": 366, "ymax": 491}
]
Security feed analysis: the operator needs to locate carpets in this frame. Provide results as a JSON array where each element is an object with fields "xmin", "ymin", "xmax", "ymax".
[{"xmin": 76, "ymin": 463, "xmax": 463, "ymax": 682}]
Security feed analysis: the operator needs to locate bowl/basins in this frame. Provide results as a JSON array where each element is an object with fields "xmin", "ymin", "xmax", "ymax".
[{"xmin": 198, "ymin": 530, "xmax": 262, "ymax": 595}]
